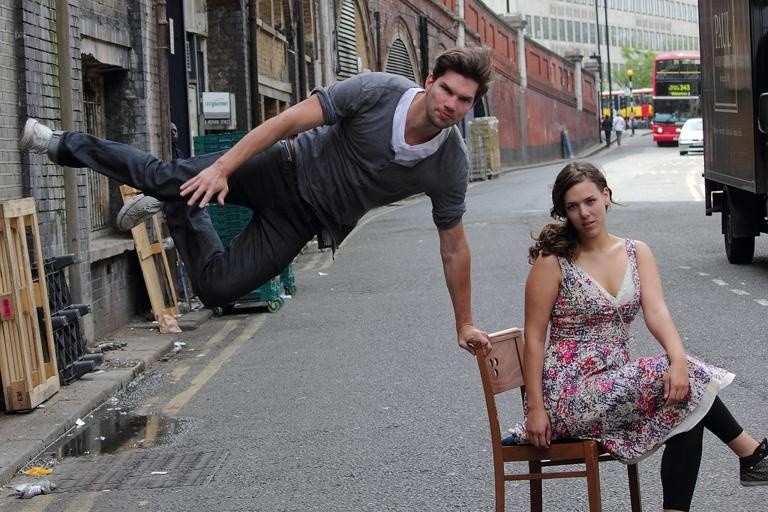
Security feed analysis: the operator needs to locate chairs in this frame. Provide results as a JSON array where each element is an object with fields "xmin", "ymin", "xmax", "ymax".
[{"xmin": 473, "ymin": 326, "xmax": 643, "ymax": 511}]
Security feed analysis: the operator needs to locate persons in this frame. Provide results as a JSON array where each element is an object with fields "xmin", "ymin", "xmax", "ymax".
[
  {"xmin": 612, "ymin": 113, "xmax": 627, "ymax": 148},
  {"xmin": 602, "ymin": 116, "xmax": 612, "ymax": 148},
  {"xmin": 18, "ymin": 45, "xmax": 493, "ymax": 359},
  {"xmin": 508, "ymin": 162, "xmax": 768, "ymax": 512}
]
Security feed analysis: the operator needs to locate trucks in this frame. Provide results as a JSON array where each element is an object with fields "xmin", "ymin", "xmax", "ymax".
[{"xmin": 699, "ymin": 0, "xmax": 768, "ymax": 263}]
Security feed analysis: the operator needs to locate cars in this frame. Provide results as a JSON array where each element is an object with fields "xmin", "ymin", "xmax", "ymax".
[{"xmin": 679, "ymin": 118, "xmax": 704, "ymax": 156}]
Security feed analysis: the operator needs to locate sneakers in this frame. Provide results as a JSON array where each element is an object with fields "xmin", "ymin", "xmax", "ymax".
[
  {"xmin": 115, "ymin": 193, "xmax": 164, "ymax": 232},
  {"xmin": 18, "ymin": 118, "xmax": 54, "ymax": 153},
  {"xmin": 740, "ymin": 438, "xmax": 768, "ymax": 486}
]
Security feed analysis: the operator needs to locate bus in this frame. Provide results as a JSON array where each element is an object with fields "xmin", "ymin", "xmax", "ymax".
[{"xmin": 599, "ymin": 51, "xmax": 701, "ymax": 147}]
